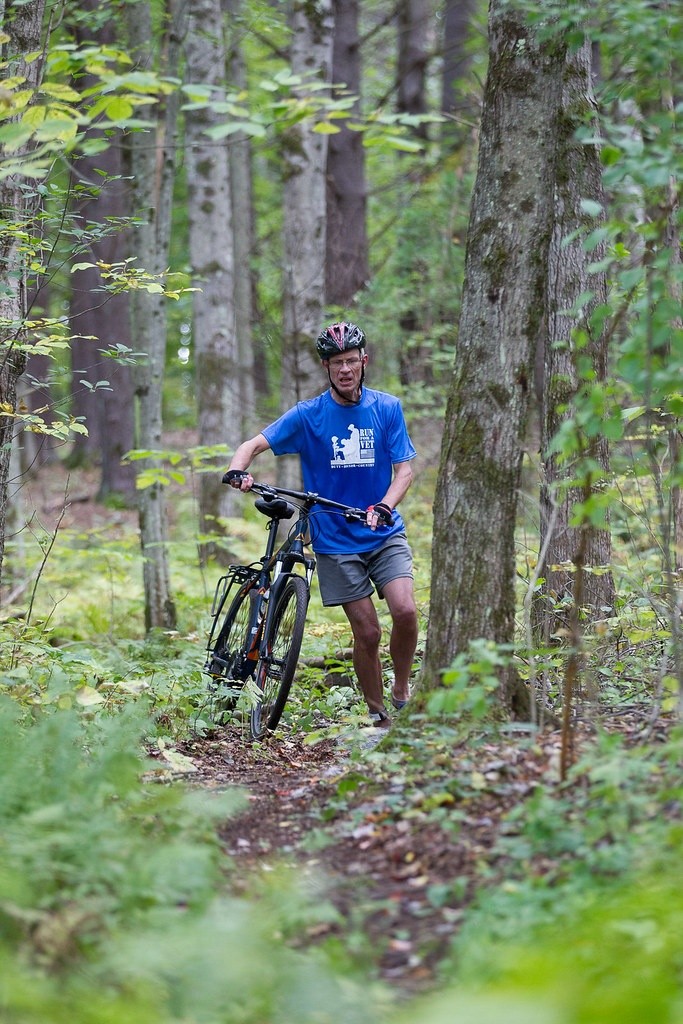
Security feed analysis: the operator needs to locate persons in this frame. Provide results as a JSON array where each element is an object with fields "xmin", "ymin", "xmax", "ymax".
[{"xmin": 222, "ymin": 322, "xmax": 418, "ymax": 729}]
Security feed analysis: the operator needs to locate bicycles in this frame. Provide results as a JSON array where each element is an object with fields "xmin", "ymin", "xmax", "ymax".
[{"xmin": 201, "ymin": 473, "xmax": 396, "ymax": 742}]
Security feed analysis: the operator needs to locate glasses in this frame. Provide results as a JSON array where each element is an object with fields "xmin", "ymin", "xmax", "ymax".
[{"xmin": 328, "ymin": 353, "xmax": 363, "ymax": 370}]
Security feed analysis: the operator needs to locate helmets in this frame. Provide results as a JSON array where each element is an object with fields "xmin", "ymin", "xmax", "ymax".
[{"xmin": 316, "ymin": 321, "xmax": 366, "ymax": 360}]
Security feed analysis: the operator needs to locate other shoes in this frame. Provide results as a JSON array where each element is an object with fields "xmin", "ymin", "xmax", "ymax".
[
  {"xmin": 369, "ymin": 707, "xmax": 393, "ymax": 728},
  {"xmin": 391, "ymin": 687, "xmax": 410, "ymax": 708}
]
